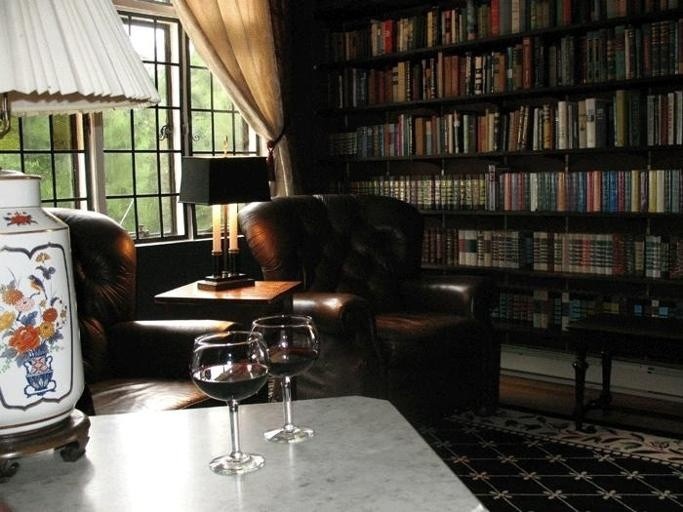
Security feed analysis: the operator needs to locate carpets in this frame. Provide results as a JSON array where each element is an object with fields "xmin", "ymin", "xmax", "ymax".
[{"xmin": 402, "ymin": 402, "xmax": 681, "ymax": 511}]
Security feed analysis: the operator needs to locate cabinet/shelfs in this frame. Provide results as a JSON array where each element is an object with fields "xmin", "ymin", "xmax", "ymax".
[{"xmin": 316, "ymin": 0, "xmax": 683, "ymax": 406}]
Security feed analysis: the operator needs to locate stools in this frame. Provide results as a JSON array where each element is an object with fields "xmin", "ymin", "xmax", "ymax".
[{"xmin": 566, "ymin": 312, "xmax": 682, "ymax": 430}]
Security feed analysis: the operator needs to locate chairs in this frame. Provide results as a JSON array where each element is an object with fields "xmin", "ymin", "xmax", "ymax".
[
  {"xmin": 40, "ymin": 206, "xmax": 245, "ymax": 414},
  {"xmin": 237, "ymin": 194, "xmax": 504, "ymax": 415}
]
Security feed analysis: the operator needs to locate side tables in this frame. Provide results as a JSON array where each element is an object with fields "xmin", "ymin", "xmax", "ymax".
[{"xmin": 152, "ymin": 279, "xmax": 302, "ymax": 403}]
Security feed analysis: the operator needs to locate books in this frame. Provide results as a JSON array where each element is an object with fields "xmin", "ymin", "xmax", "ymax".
[
  {"xmin": 344, "ymin": 167, "xmax": 683, "ymax": 214},
  {"xmin": 416, "ymin": 226, "xmax": 683, "ymax": 280},
  {"xmin": 336, "ymin": 1, "xmax": 683, "ymax": 160},
  {"xmin": 473, "ymin": 283, "xmax": 683, "ymax": 331}
]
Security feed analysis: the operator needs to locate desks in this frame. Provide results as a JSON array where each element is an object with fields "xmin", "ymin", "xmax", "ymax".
[{"xmin": 0, "ymin": 395, "xmax": 491, "ymax": 512}]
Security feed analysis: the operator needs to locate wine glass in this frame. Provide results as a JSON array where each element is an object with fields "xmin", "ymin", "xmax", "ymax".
[{"xmin": 193, "ymin": 313, "xmax": 323, "ymax": 476}]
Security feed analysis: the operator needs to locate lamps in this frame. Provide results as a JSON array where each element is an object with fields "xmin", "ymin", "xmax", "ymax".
[
  {"xmin": 177, "ymin": 157, "xmax": 272, "ymax": 293},
  {"xmin": 0, "ymin": 0, "xmax": 160, "ymax": 483}
]
